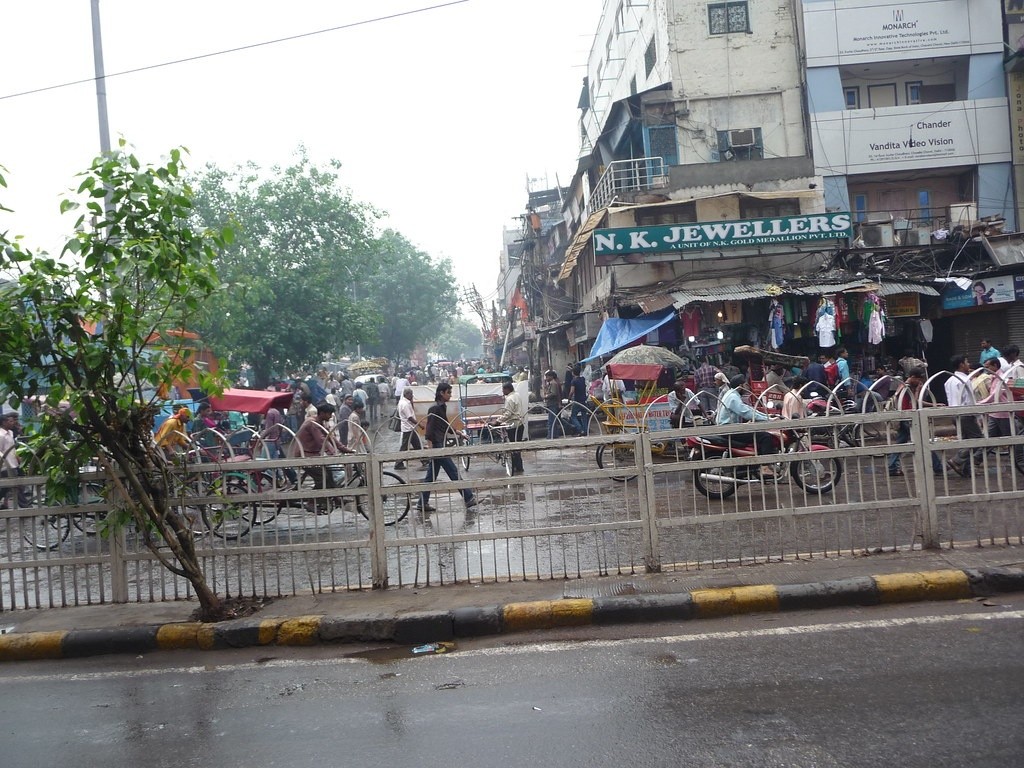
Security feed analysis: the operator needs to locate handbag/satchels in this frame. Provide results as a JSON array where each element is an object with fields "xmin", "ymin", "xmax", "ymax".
[
  {"xmin": 353, "ymin": 391, "xmax": 364, "ymax": 409},
  {"xmin": 388, "ymin": 410, "xmax": 401, "ymax": 432}
]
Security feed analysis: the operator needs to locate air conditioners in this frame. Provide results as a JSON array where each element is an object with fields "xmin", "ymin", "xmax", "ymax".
[
  {"xmin": 725, "ymin": 128, "xmax": 756, "ymax": 147},
  {"xmin": 897, "ymin": 228, "xmax": 931, "ymax": 246},
  {"xmin": 861, "ymin": 225, "xmax": 894, "ymax": 247}
]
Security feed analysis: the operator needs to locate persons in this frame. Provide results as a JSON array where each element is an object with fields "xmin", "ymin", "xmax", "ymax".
[
  {"xmin": 221, "ymin": 367, "xmax": 485, "ymax": 512},
  {"xmin": 601, "ymin": 337, "xmax": 891, "ymax": 479},
  {"xmin": 888, "ymin": 367, "xmax": 953, "ymax": 475},
  {"xmin": 979, "ymin": 337, "xmax": 1001, "ymax": 366},
  {"xmin": 973, "ymin": 281, "xmax": 995, "ymax": 305},
  {"xmin": 0, "ymin": 395, "xmax": 219, "ymax": 507},
  {"xmin": 1003, "ymin": 344, "xmax": 1023, "ymax": 378},
  {"xmin": 944, "ymin": 354, "xmax": 985, "ymax": 476},
  {"xmin": 972, "ymin": 357, "xmax": 1020, "ymax": 465},
  {"xmin": 399, "ymin": 359, "xmax": 587, "ymax": 473}
]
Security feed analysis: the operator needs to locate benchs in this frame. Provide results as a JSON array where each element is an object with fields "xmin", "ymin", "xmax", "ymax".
[
  {"xmin": 209, "ymin": 454, "xmax": 251, "ymax": 466},
  {"xmin": 459, "ymin": 396, "xmax": 506, "ymax": 421},
  {"xmin": 590, "ymin": 395, "xmax": 648, "ymax": 429}
]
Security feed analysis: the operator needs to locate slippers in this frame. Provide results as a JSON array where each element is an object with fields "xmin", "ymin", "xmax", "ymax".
[{"xmin": 758, "ymin": 469, "xmax": 780, "ymax": 480}]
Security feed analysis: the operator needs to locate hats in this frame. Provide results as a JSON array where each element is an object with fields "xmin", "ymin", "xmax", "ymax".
[
  {"xmin": 715, "ymin": 372, "xmax": 730, "ymax": 384},
  {"xmin": 730, "ymin": 374, "xmax": 746, "ymax": 387},
  {"xmin": 174, "ymin": 408, "xmax": 193, "ymax": 418},
  {"xmin": 344, "ymin": 394, "xmax": 352, "ymax": 401}
]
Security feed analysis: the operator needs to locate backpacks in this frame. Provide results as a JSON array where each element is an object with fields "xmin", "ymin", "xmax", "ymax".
[
  {"xmin": 824, "ymin": 359, "xmax": 845, "ymax": 388},
  {"xmin": 883, "ymin": 384, "xmax": 911, "ymax": 431}
]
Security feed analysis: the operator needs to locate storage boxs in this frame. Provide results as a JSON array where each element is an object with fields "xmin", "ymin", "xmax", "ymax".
[{"xmin": 945, "ymin": 202, "xmax": 978, "ymax": 221}]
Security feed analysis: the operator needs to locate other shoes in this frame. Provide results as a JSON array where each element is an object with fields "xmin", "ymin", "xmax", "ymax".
[
  {"xmin": 890, "ymin": 469, "xmax": 904, "ymax": 476},
  {"xmin": 969, "ymin": 463, "xmax": 981, "ymax": 475},
  {"xmin": 304, "ymin": 503, "xmax": 321, "ymax": 515},
  {"xmin": 947, "ymin": 459, "xmax": 967, "ymax": 478},
  {"xmin": 416, "ymin": 504, "xmax": 435, "ymax": 511},
  {"xmin": 934, "ymin": 465, "xmax": 950, "ymax": 475},
  {"xmin": 421, "ymin": 460, "xmax": 430, "ymax": 465},
  {"xmin": 466, "ymin": 495, "xmax": 486, "ymax": 508},
  {"xmin": 573, "ymin": 431, "xmax": 584, "ymax": 437},
  {"xmin": 394, "ymin": 464, "xmax": 406, "ymax": 469}
]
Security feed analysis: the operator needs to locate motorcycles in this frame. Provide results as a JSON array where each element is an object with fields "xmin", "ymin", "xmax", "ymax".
[{"xmin": 683, "ymin": 401, "xmax": 843, "ymax": 499}]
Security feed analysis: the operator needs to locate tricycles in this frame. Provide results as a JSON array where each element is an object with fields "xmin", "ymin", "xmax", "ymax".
[
  {"xmin": 594, "ymin": 343, "xmax": 712, "ymax": 483},
  {"xmin": 732, "ymin": 345, "xmax": 895, "ymax": 458},
  {"xmin": 460, "ymin": 372, "xmax": 520, "ymax": 476},
  {"xmin": 0, "ymin": 379, "xmax": 471, "ymax": 553}
]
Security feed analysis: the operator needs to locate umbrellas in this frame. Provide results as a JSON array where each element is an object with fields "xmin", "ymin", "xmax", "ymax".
[
  {"xmin": 599, "ymin": 342, "xmax": 686, "ymax": 370},
  {"xmin": 347, "ymin": 361, "xmax": 383, "ymax": 371}
]
